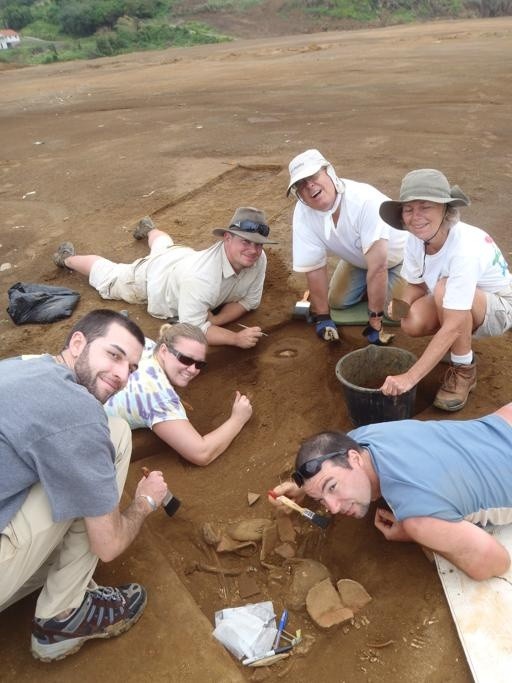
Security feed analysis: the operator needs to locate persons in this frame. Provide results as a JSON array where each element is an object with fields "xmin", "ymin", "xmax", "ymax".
[
  {"xmin": 285, "ymin": 149, "xmax": 407, "ymax": 344},
  {"xmin": 54, "ymin": 206, "xmax": 278, "ymax": 349},
  {"xmin": 269, "ymin": 402, "xmax": 512, "ymax": 581},
  {"xmin": 0, "ymin": 310, "xmax": 169, "ymax": 663},
  {"xmin": 6, "ymin": 323, "xmax": 252, "ymax": 467},
  {"xmin": 379, "ymin": 169, "xmax": 512, "ymax": 412}
]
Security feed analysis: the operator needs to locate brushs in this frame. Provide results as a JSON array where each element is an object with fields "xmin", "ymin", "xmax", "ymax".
[
  {"xmin": 141, "ymin": 466, "xmax": 180, "ymax": 517},
  {"xmin": 293, "ymin": 290, "xmax": 311, "ymax": 317},
  {"xmin": 268, "ymin": 489, "xmax": 331, "ymax": 529}
]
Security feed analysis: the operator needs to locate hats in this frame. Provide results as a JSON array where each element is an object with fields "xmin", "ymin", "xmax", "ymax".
[
  {"xmin": 213, "ymin": 207, "xmax": 280, "ymax": 244},
  {"xmin": 286, "ymin": 149, "xmax": 344, "ymax": 213},
  {"xmin": 379, "ymin": 169, "xmax": 470, "ymax": 230}
]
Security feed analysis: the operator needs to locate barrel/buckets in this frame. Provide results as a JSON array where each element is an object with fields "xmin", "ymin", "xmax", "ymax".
[{"xmin": 336, "ymin": 345, "xmax": 420, "ymax": 430}]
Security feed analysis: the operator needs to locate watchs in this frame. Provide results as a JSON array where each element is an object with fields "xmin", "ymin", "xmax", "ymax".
[{"xmin": 135, "ymin": 494, "xmax": 155, "ymax": 511}]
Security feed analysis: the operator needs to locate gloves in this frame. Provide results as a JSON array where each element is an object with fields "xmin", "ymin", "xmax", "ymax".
[
  {"xmin": 363, "ymin": 320, "xmax": 396, "ymax": 346},
  {"xmin": 308, "ymin": 303, "xmax": 340, "ymax": 344}
]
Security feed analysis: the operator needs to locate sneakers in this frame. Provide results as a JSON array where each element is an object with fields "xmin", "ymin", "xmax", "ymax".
[
  {"xmin": 432, "ymin": 352, "xmax": 478, "ymax": 412},
  {"xmin": 31, "ymin": 582, "xmax": 148, "ymax": 663}
]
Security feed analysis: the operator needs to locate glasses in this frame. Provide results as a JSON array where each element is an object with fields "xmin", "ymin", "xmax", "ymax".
[
  {"xmin": 230, "ymin": 220, "xmax": 270, "ymax": 237},
  {"xmin": 291, "ymin": 449, "xmax": 347, "ymax": 489},
  {"xmin": 165, "ymin": 340, "xmax": 208, "ymax": 370}
]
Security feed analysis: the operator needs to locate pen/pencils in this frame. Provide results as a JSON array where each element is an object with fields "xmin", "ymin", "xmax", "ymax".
[
  {"xmin": 242, "ymin": 645, "xmax": 295, "ymax": 666},
  {"xmin": 274, "ymin": 609, "xmax": 288, "ymax": 650},
  {"xmin": 237, "ymin": 323, "xmax": 268, "ymax": 337}
]
